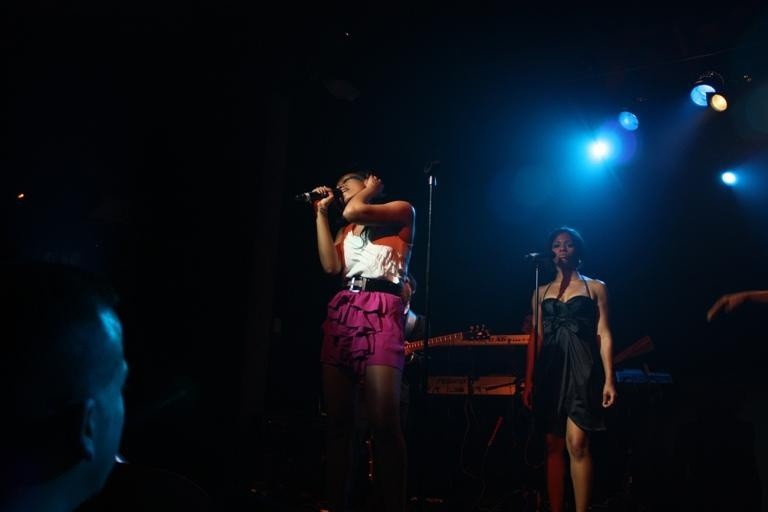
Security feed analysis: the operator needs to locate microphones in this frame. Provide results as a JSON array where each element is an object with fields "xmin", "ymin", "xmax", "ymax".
[
  {"xmin": 524, "ymin": 249, "xmax": 558, "ymax": 261},
  {"xmin": 296, "ymin": 187, "xmax": 342, "ymax": 202}
]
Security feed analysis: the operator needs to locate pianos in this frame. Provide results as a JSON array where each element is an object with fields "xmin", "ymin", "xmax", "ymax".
[{"xmin": 425, "ymin": 376, "xmax": 518, "ymax": 396}]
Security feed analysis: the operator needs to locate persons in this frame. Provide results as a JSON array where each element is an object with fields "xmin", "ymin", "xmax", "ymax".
[
  {"xmin": 309, "ymin": 170, "xmax": 418, "ymax": 511},
  {"xmin": 704, "ymin": 287, "xmax": 767, "ymax": 326},
  {"xmin": 519, "ymin": 224, "xmax": 616, "ymax": 512},
  {"xmin": 1, "ymin": 268, "xmax": 130, "ymax": 511}
]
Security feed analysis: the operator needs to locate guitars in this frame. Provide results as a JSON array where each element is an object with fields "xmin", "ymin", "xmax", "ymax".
[{"xmin": 404, "ymin": 324, "xmax": 490, "ymax": 356}]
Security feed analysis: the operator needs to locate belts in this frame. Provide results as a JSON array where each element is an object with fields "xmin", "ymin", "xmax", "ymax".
[{"xmin": 344, "ymin": 275, "xmax": 401, "ymax": 296}]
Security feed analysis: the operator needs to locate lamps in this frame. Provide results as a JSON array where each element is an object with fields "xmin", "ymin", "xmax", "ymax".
[
  {"xmin": 717, "ymin": 169, "xmax": 736, "ymax": 193},
  {"xmin": 619, "ymin": 94, "xmax": 648, "ymax": 131},
  {"xmin": 689, "ymin": 67, "xmax": 732, "ymax": 115}
]
